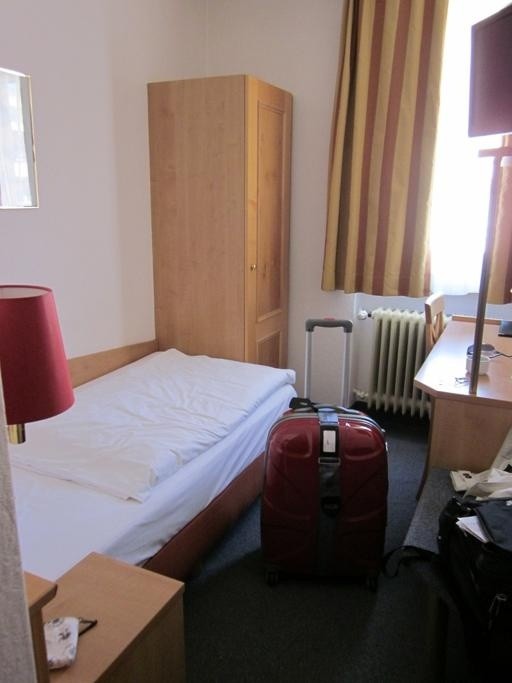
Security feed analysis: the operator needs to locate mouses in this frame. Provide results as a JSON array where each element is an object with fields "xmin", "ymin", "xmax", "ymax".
[{"xmin": 468, "ymin": 343, "xmax": 496, "ymax": 355}]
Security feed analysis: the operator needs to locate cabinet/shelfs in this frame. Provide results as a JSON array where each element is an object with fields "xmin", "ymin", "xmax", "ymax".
[{"xmin": 146, "ymin": 75, "xmax": 294, "ymax": 367}]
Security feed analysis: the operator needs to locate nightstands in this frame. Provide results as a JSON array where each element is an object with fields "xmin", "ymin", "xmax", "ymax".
[{"xmin": 41, "ymin": 550, "xmax": 185, "ymax": 683}]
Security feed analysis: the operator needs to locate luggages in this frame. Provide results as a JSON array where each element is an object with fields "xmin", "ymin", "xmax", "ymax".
[{"xmin": 261, "ymin": 317, "xmax": 388, "ymax": 590}]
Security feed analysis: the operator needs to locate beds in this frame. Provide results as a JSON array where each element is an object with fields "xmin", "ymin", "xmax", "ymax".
[{"xmin": 8, "ymin": 349, "xmax": 298, "ymax": 583}]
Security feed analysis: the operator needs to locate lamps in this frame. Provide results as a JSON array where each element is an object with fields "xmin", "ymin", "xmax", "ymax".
[{"xmin": 0, "ymin": 285, "xmax": 76, "ymax": 445}]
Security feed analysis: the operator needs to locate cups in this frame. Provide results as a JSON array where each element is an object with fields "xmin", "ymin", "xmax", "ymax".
[{"xmin": 465, "ymin": 353, "xmax": 490, "ymax": 375}]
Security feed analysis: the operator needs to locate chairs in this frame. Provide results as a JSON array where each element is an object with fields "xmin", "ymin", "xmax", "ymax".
[{"xmin": 424, "ymin": 286, "xmax": 444, "ymax": 353}]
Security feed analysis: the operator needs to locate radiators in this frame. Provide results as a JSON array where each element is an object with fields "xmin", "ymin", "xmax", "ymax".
[{"xmin": 352, "ymin": 308, "xmax": 451, "ymax": 420}]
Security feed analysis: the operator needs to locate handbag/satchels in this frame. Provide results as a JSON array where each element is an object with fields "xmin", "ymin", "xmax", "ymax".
[{"xmin": 437, "ymin": 496, "xmax": 512, "ymax": 631}]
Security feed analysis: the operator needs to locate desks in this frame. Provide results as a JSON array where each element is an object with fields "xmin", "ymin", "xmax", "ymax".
[{"xmin": 413, "ymin": 320, "xmax": 511, "ymax": 500}]
